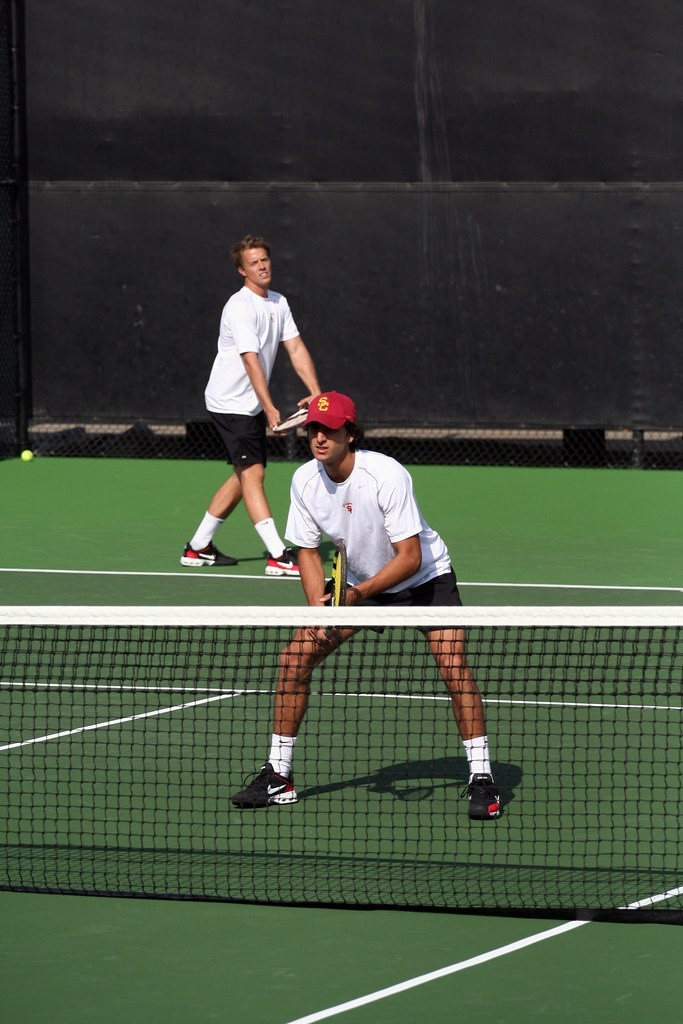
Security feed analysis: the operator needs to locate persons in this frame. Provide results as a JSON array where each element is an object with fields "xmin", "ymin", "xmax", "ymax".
[
  {"xmin": 231, "ymin": 390, "xmax": 502, "ymax": 821},
  {"xmin": 181, "ymin": 234, "xmax": 321, "ymax": 576}
]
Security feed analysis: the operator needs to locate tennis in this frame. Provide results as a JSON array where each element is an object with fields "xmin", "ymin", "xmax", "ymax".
[{"xmin": 21, "ymin": 450, "xmax": 33, "ymax": 462}]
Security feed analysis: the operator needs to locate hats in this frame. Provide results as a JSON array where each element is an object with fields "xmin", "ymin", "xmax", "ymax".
[{"xmin": 303, "ymin": 390, "xmax": 357, "ymax": 429}]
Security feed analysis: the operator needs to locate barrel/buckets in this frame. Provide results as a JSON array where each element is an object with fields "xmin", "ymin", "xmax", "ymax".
[{"xmin": 562, "ymin": 424, "xmax": 607, "ymax": 466}]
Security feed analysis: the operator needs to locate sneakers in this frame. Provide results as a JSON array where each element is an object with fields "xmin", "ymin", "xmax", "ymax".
[
  {"xmin": 179, "ymin": 541, "xmax": 238, "ymax": 566},
  {"xmin": 265, "ymin": 546, "xmax": 300, "ymax": 575},
  {"xmin": 459, "ymin": 773, "xmax": 501, "ymax": 817},
  {"xmin": 231, "ymin": 763, "xmax": 298, "ymax": 804}
]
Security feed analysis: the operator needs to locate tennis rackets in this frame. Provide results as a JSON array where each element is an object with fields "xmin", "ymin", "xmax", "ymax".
[
  {"xmin": 272, "ymin": 405, "xmax": 308, "ymax": 434},
  {"xmin": 325, "ymin": 543, "xmax": 348, "ymax": 640}
]
